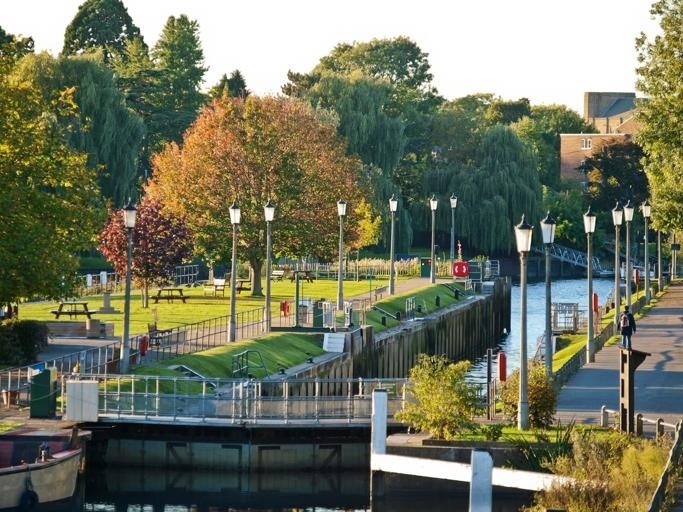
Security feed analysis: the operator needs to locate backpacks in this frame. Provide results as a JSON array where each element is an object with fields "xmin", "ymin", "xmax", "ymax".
[{"xmin": 620, "ymin": 312, "xmax": 630, "ymax": 327}]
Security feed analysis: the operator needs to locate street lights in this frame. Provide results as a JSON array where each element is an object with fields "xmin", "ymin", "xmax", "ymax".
[
  {"xmin": 388, "ymin": 194, "xmax": 400, "ymax": 295},
  {"xmin": 639, "ymin": 199, "xmax": 651, "ymax": 298},
  {"xmin": 624, "ymin": 195, "xmax": 633, "ymax": 320},
  {"xmin": 429, "ymin": 194, "xmax": 437, "ymax": 281},
  {"xmin": 119, "ymin": 202, "xmax": 137, "ymax": 372},
  {"xmin": 335, "ymin": 196, "xmax": 347, "ymax": 311},
  {"xmin": 261, "ymin": 199, "xmax": 277, "ymax": 333},
  {"xmin": 449, "ymin": 193, "xmax": 457, "ymax": 280},
  {"xmin": 581, "ymin": 202, "xmax": 598, "ymax": 364},
  {"xmin": 512, "ymin": 212, "xmax": 531, "ymax": 429},
  {"xmin": 539, "ymin": 209, "xmax": 557, "ymax": 376},
  {"xmin": 609, "ymin": 199, "xmax": 623, "ymax": 336},
  {"xmin": 228, "ymin": 201, "xmax": 240, "ymax": 341}
]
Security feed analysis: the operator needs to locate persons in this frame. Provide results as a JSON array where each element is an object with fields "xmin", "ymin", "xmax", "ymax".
[
  {"xmin": 36, "ymin": 444, "xmax": 58, "ymax": 464},
  {"xmin": 12, "ymin": 306, "xmax": 18, "ymax": 316},
  {"xmin": 617, "ymin": 306, "xmax": 636, "ymax": 348},
  {"xmin": 4, "ymin": 302, "xmax": 12, "ymax": 319}
]
